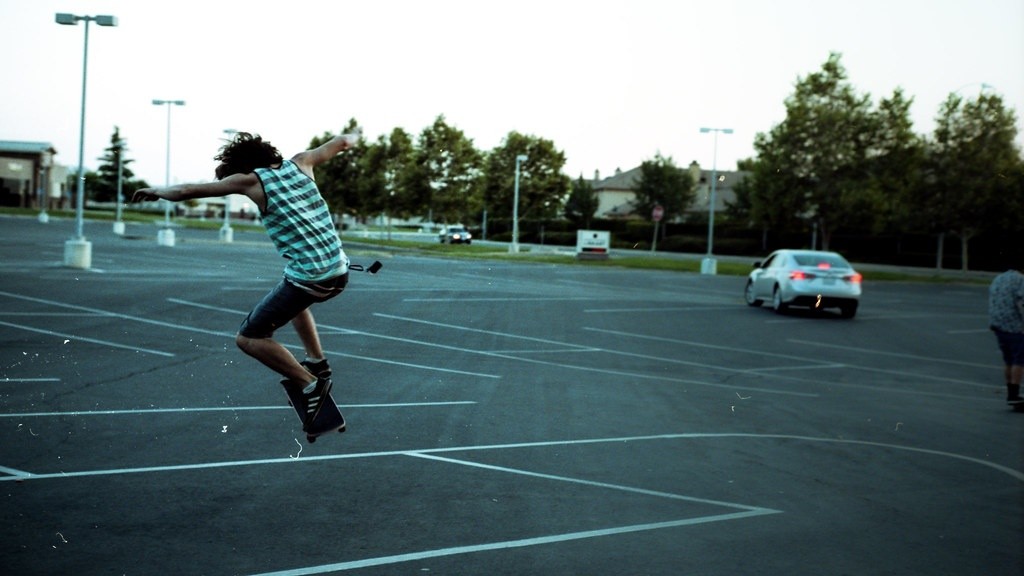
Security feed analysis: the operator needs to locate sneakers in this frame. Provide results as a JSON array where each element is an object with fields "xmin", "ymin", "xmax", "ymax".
[
  {"xmin": 305, "ymin": 378, "xmax": 334, "ymax": 421},
  {"xmin": 299, "ymin": 359, "xmax": 332, "ymax": 378}
]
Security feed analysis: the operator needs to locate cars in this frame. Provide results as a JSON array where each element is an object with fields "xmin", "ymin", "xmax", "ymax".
[
  {"xmin": 744, "ymin": 249, "xmax": 863, "ymax": 319},
  {"xmin": 437, "ymin": 226, "xmax": 472, "ymax": 245}
]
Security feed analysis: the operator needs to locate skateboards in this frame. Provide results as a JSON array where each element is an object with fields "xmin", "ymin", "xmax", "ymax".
[
  {"xmin": 279, "ymin": 378, "xmax": 347, "ymax": 444},
  {"xmin": 1007, "ymin": 400, "xmax": 1024, "ymax": 409}
]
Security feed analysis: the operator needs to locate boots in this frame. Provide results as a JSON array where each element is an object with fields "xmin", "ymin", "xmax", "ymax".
[{"xmin": 1005, "ymin": 382, "xmax": 1024, "ymax": 405}]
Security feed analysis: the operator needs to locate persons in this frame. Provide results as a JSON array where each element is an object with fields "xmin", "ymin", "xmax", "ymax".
[
  {"xmin": 988, "ymin": 257, "xmax": 1024, "ymax": 413},
  {"xmin": 131, "ymin": 131, "xmax": 356, "ymax": 429}
]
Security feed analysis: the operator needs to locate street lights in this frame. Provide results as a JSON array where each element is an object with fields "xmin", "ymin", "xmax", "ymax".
[
  {"xmin": 509, "ymin": 154, "xmax": 530, "ymax": 258},
  {"xmin": 698, "ymin": 126, "xmax": 732, "ymax": 275},
  {"xmin": 220, "ymin": 129, "xmax": 240, "ymax": 244},
  {"xmin": 151, "ymin": 98, "xmax": 186, "ymax": 248},
  {"xmin": 112, "ymin": 139, "xmax": 127, "ymax": 236},
  {"xmin": 55, "ymin": 12, "xmax": 118, "ymax": 273}
]
